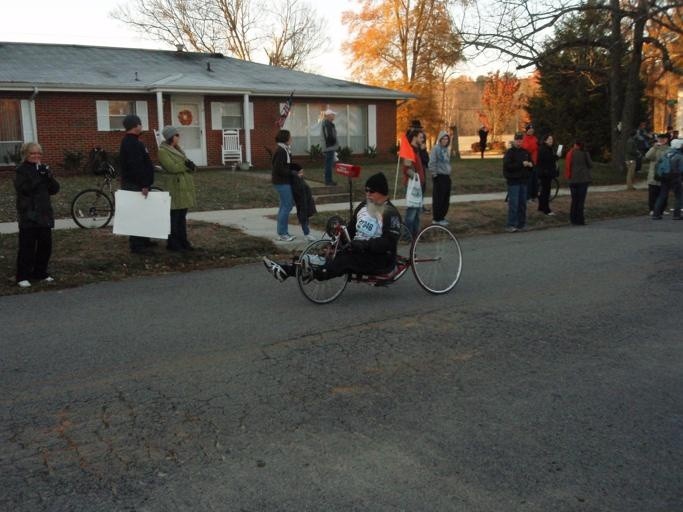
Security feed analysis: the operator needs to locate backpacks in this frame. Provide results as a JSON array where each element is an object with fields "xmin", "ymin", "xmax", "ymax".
[{"xmin": 653, "ymin": 151, "xmax": 677, "ymax": 183}]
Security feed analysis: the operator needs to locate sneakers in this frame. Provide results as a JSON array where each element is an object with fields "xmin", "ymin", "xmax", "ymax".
[
  {"xmin": 431, "ymin": 220, "xmax": 448, "ymax": 225},
  {"xmin": 277, "ymin": 234, "xmax": 293, "ymax": 242},
  {"xmin": 504, "ymin": 227, "xmax": 517, "ymax": 233},
  {"xmin": 43, "ymin": 276, "xmax": 54, "ymax": 282},
  {"xmin": 263, "ymin": 256, "xmax": 289, "ymax": 284},
  {"xmin": 301, "ymin": 255, "xmax": 314, "ymax": 285},
  {"xmin": 17, "ymin": 280, "xmax": 32, "ymax": 288}
]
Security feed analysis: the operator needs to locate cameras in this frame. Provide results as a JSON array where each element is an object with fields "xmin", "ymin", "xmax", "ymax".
[{"xmin": 656, "ymin": 142, "xmax": 662, "ymax": 147}]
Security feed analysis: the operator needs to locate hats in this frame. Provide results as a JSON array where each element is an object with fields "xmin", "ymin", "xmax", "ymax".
[
  {"xmin": 290, "ymin": 163, "xmax": 302, "ymax": 172},
  {"xmin": 162, "ymin": 127, "xmax": 178, "ymax": 140},
  {"xmin": 513, "ymin": 132, "xmax": 522, "ymax": 139},
  {"xmin": 670, "ymin": 139, "xmax": 682, "ymax": 150},
  {"xmin": 365, "ymin": 172, "xmax": 388, "ymax": 196},
  {"xmin": 325, "ymin": 109, "xmax": 336, "ymax": 116},
  {"xmin": 408, "ymin": 120, "xmax": 424, "ymax": 128}
]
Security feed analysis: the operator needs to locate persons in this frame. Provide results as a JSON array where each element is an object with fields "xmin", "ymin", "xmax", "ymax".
[
  {"xmin": 271, "ymin": 130, "xmax": 304, "ymax": 242},
  {"xmin": 626, "ymin": 119, "xmax": 683, "ymax": 220},
  {"xmin": 503, "ymin": 124, "xmax": 594, "ymax": 232},
  {"xmin": 16, "ymin": 143, "xmax": 61, "ymax": 288},
  {"xmin": 262, "ymin": 171, "xmax": 402, "ymax": 283},
  {"xmin": 156, "ymin": 125, "xmax": 196, "ymax": 252},
  {"xmin": 479, "ymin": 126, "xmax": 489, "ymax": 159},
  {"xmin": 119, "ymin": 115, "xmax": 157, "ymax": 256},
  {"xmin": 403, "ymin": 120, "xmax": 452, "ymax": 240},
  {"xmin": 288, "ymin": 163, "xmax": 318, "ymax": 242},
  {"xmin": 322, "ymin": 109, "xmax": 340, "ymax": 185}
]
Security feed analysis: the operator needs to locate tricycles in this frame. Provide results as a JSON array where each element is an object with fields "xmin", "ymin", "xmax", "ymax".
[{"xmin": 294, "ymin": 210, "xmax": 464, "ymax": 306}]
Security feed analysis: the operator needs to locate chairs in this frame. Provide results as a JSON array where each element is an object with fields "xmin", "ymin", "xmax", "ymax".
[{"xmin": 220, "ymin": 127, "xmax": 243, "ymax": 166}]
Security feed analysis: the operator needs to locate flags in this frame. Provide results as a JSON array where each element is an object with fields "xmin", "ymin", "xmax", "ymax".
[
  {"xmin": 399, "ymin": 133, "xmax": 416, "ymax": 163},
  {"xmin": 272, "ymin": 93, "xmax": 293, "ymax": 127}
]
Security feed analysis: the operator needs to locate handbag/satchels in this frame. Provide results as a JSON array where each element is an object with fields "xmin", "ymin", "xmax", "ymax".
[{"xmin": 405, "ymin": 174, "xmax": 423, "ymax": 209}]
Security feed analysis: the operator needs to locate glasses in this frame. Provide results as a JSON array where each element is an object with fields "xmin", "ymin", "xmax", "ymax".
[{"xmin": 366, "ymin": 188, "xmax": 375, "ymax": 193}]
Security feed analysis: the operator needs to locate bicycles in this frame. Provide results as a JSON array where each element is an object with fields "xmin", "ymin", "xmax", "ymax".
[
  {"xmin": 504, "ymin": 168, "xmax": 564, "ymax": 202},
  {"xmin": 71, "ymin": 160, "xmax": 165, "ymax": 229}
]
